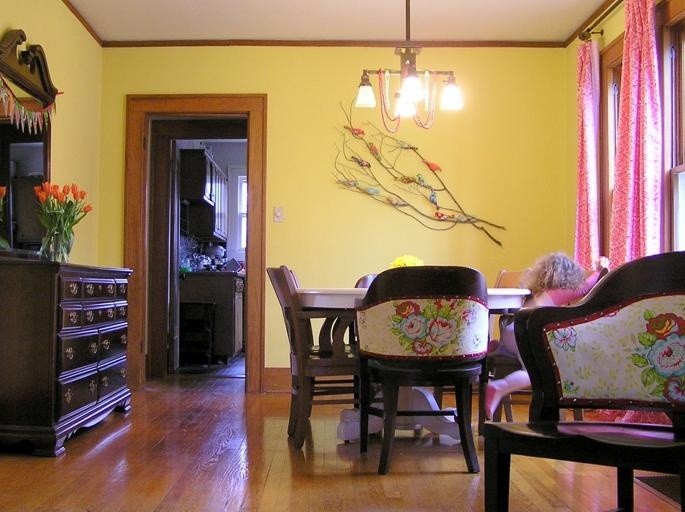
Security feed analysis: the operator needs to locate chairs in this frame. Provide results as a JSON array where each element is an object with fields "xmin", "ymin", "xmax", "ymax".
[
  {"xmin": 355, "ymin": 266, "xmax": 488, "ymax": 475},
  {"xmin": 349, "ymin": 274, "xmax": 444, "ymax": 441},
  {"xmin": 276, "ymin": 265, "xmax": 361, "ymax": 449},
  {"xmin": 267, "ymin": 267, "xmax": 357, "ymax": 436},
  {"xmin": 489, "ymin": 268, "xmax": 531, "ymax": 423},
  {"xmin": 479, "ymin": 250, "xmax": 685, "ymax": 512}
]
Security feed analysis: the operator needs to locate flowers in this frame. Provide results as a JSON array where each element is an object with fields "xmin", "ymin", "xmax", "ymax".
[
  {"xmin": 0, "ymin": 187, "xmax": 8, "ymax": 248},
  {"xmin": 387, "ymin": 299, "xmax": 479, "ymax": 357},
  {"xmin": 627, "ymin": 308, "xmax": 685, "ymax": 404},
  {"xmin": 552, "ymin": 329, "xmax": 579, "ymax": 351},
  {"xmin": 33, "ymin": 181, "xmax": 92, "ymax": 262}
]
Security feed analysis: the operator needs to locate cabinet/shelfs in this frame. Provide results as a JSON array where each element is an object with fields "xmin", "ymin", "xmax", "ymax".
[
  {"xmin": 178, "ymin": 148, "xmax": 215, "ymax": 207},
  {"xmin": 190, "ymin": 166, "xmax": 229, "ymax": 243},
  {"xmin": 176, "ymin": 271, "xmax": 246, "ymax": 366},
  {"xmin": 0, "ymin": 260, "xmax": 132, "ymax": 457}
]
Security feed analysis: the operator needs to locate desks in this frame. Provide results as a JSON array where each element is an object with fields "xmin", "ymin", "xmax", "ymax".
[{"xmin": 297, "ymin": 287, "xmax": 528, "ymax": 440}]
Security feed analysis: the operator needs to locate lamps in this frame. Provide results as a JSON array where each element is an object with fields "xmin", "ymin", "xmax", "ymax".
[{"xmin": 355, "ymin": 1, "xmax": 462, "ymax": 117}]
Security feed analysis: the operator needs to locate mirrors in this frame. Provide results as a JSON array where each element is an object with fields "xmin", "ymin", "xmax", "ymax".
[{"xmin": 0, "ymin": 28, "xmax": 57, "ymax": 259}]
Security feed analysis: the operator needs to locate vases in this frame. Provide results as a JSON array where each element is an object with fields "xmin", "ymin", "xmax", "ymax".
[{"xmin": 42, "ymin": 232, "xmax": 73, "ymax": 262}]
